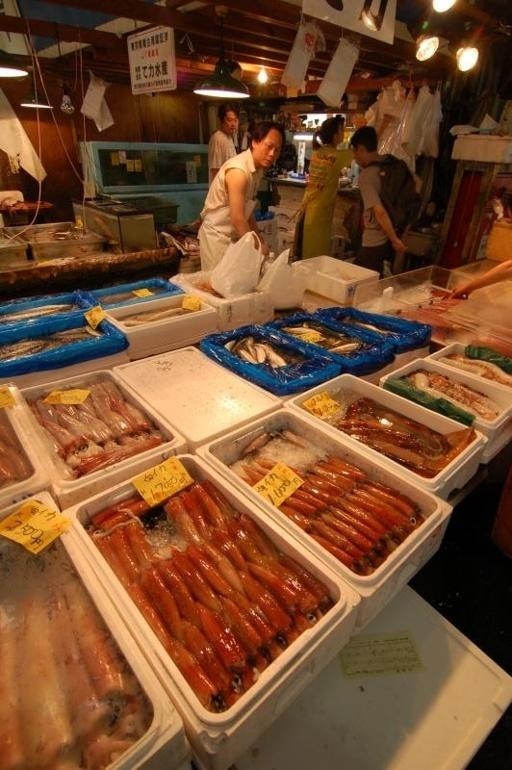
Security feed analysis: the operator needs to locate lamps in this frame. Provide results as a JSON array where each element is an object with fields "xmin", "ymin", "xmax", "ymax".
[
  {"xmin": 361, "ymin": 0, "xmax": 479, "ymax": 73},
  {"xmin": 0, "ymin": 22, "xmax": 75, "ymax": 115},
  {"xmin": 193, "ymin": 14, "xmax": 251, "ymax": 101}
]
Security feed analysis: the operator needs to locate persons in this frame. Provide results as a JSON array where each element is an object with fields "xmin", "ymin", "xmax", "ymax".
[
  {"xmin": 343, "ymin": 125, "xmax": 424, "ymax": 276},
  {"xmin": 197, "ymin": 120, "xmax": 285, "ymax": 278},
  {"xmin": 338, "ymin": 140, "xmax": 362, "ymax": 187},
  {"xmin": 206, "ymin": 104, "xmax": 240, "ymax": 194},
  {"xmin": 287, "ymin": 113, "xmax": 357, "ymax": 263}
]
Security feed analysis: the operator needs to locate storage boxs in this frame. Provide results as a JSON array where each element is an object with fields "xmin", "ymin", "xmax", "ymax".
[
  {"xmin": 109, "ymin": 347, "xmax": 284, "ymax": 442},
  {"xmin": 382, "ymin": 356, "xmax": 511, "ymax": 464},
  {"xmin": 1, "ymin": 255, "xmax": 383, "ymax": 378},
  {"xmin": 2, "ymin": 382, "xmax": 52, "ymax": 508},
  {"xmin": 13, "ymin": 368, "xmax": 194, "ymax": 510},
  {"xmin": 303, "ymin": 300, "xmax": 434, "ymax": 365},
  {"xmin": 56, "ymin": 454, "xmax": 363, "ymax": 769},
  {"xmin": 200, "ymin": 325, "xmax": 335, "ymax": 395},
  {"xmin": 0, "ymin": 488, "xmax": 192, "ymax": 770},
  {"xmin": 196, "ymin": 410, "xmax": 452, "ymax": 636},
  {"xmin": 266, "ymin": 314, "xmax": 394, "ymax": 371},
  {"xmin": 427, "ymin": 344, "xmax": 511, "ymax": 386},
  {"xmin": 289, "ymin": 374, "xmax": 485, "ymax": 518}
]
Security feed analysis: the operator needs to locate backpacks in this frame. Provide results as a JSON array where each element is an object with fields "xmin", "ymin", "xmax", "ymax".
[{"xmin": 363, "ymin": 153, "xmax": 421, "ymax": 233}]
